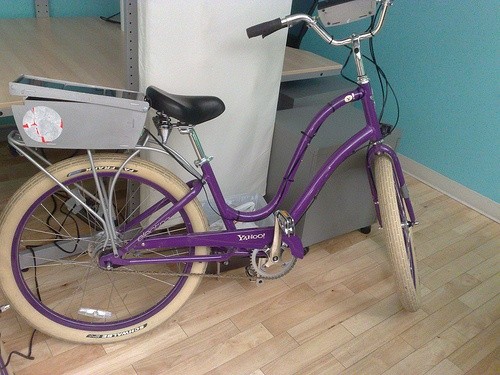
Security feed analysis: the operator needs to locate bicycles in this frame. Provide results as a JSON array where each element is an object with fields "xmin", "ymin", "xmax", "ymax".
[{"xmin": 1, "ymin": 0, "xmax": 420, "ymax": 342}]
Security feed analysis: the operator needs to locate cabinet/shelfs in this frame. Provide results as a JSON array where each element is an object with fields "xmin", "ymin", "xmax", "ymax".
[{"xmin": 265, "ymin": 77, "xmax": 378, "ymax": 254}]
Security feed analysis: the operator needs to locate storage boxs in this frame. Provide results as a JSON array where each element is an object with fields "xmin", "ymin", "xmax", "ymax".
[{"xmin": 9, "ymin": 73, "xmax": 150, "ymax": 150}]
